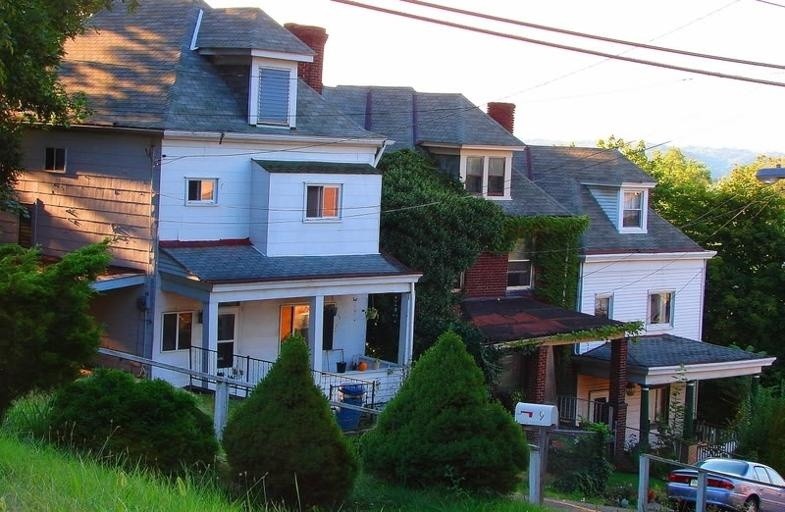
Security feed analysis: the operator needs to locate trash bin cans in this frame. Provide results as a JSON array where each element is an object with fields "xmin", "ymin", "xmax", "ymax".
[{"xmin": 336, "ymin": 383, "xmax": 367, "ymax": 433}]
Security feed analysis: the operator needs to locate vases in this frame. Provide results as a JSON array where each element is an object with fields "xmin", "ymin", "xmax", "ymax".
[
  {"xmin": 336, "ymin": 360, "xmax": 347, "ymax": 373},
  {"xmin": 358, "ymin": 361, "xmax": 367, "ymax": 371},
  {"xmin": 372, "ymin": 362, "xmax": 380, "ymax": 370}
]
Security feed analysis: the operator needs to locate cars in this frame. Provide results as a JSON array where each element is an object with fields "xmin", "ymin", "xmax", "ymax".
[{"xmin": 666, "ymin": 456, "xmax": 785, "ymax": 512}]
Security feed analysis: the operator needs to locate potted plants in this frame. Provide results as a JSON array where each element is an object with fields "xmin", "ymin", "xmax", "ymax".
[{"xmin": 364, "ymin": 306, "xmax": 380, "ymax": 322}]
